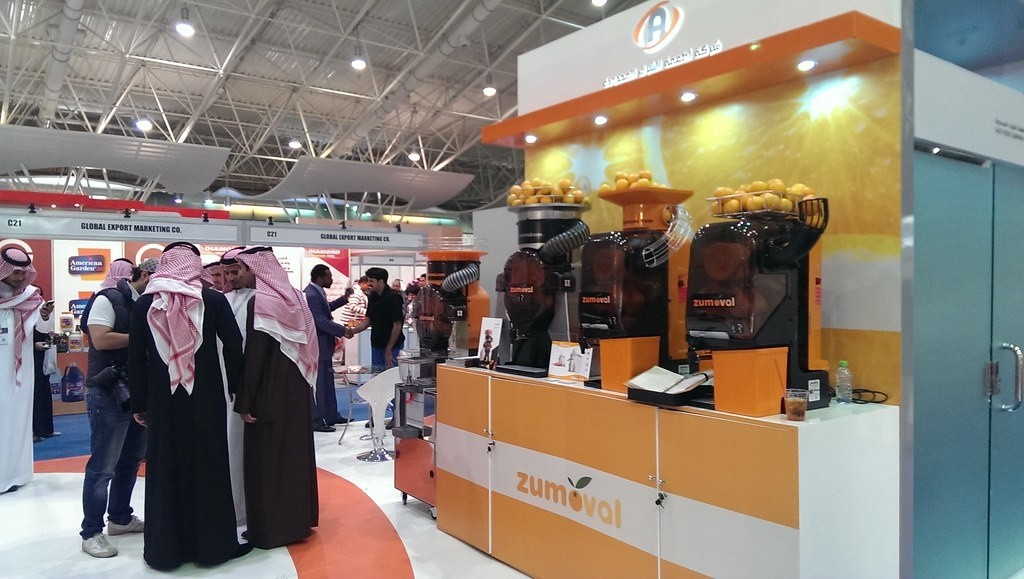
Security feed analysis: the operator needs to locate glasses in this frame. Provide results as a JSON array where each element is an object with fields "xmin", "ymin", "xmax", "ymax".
[
  {"xmin": 393, "ymin": 285, "xmax": 399, "ymax": 288},
  {"xmin": 419, "ymin": 280, "xmax": 425, "ymax": 282}
]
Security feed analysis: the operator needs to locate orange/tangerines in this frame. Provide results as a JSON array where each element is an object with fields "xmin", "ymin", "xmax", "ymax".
[
  {"xmin": 711, "ymin": 179, "xmax": 824, "ymax": 228},
  {"xmin": 508, "ymin": 177, "xmax": 584, "ymax": 205},
  {"xmin": 599, "ymin": 171, "xmax": 669, "ymax": 192}
]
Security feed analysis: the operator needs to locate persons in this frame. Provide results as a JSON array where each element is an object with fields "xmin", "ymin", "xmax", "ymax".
[
  {"xmin": 0, "ymin": 245, "xmax": 54, "ymax": 495},
  {"xmin": 31, "ymin": 241, "xmax": 427, "ymax": 569},
  {"xmin": 347, "ymin": 267, "xmax": 405, "ymax": 429},
  {"xmin": 302, "ymin": 264, "xmax": 354, "ymax": 432}
]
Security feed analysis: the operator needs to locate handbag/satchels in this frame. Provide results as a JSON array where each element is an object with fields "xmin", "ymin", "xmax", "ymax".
[{"xmin": 43, "ymin": 344, "xmax": 57, "ymax": 376}]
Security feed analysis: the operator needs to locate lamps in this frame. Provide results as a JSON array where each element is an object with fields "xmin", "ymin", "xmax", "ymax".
[
  {"xmin": 408, "ymin": 144, "xmax": 421, "ymax": 161},
  {"xmin": 175, "ymin": 1, "xmax": 195, "ymax": 38},
  {"xmin": 174, "ymin": 192, "xmax": 182, "ymax": 204},
  {"xmin": 288, "ymin": 105, "xmax": 301, "ymax": 149},
  {"xmin": 482, "ymin": 72, "xmax": 495, "ymax": 96},
  {"xmin": 203, "ymin": 187, "xmax": 213, "ymax": 205},
  {"xmin": 351, "ymin": 26, "xmax": 368, "ymax": 70},
  {"xmin": 135, "ymin": 67, "xmax": 152, "ymax": 131},
  {"xmin": 224, "ymin": 195, "xmax": 231, "ymax": 209}
]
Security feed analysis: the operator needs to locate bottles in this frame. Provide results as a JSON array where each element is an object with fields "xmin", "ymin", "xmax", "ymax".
[
  {"xmin": 48, "ymin": 367, "xmax": 61, "ymax": 400},
  {"xmin": 61, "ymin": 363, "xmax": 85, "ymax": 402},
  {"xmin": 59, "ymin": 312, "xmax": 75, "ymax": 331},
  {"xmin": 835, "ymin": 360, "xmax": 852, "ymax": 405},
  {"xmin": 54, "ymin": 331, "xmax": 90, "ymax": 353}
]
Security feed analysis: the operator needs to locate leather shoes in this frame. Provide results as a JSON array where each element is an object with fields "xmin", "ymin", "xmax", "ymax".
[
  {"xmin": 228, "ymin": 542, "xmax": 254, "ymax": 559},
  {"xmin": 327, "ymin": 417, "xmax": 354, "ymax": 426},
  {"xmin": 313, "ymin": 426, "xmax": 336, "ymax": 432}
]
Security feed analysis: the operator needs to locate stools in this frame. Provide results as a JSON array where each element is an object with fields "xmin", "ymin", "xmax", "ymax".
[{"xmin": 357, "ymin": 365, "xmax": 404, "ymax": 463}]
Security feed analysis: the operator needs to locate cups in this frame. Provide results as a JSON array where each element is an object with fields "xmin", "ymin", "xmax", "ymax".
[{"xmin": 783, "ymin": 389, "xmax": 809, "ymax": 421}]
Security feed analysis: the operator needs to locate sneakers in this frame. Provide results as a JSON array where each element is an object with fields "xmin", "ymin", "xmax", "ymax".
[
  {"xmin": 107, "ymin": 515, "xmax": 145, "ymax": 535},
  {"xmin": 82, "ymin": 533, "xmax": 118, "ymax": 558}
]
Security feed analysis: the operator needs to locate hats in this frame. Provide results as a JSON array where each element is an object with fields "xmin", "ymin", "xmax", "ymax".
[{"xmin": 138, "ymin": 258, "xmax": 160, "ymax": 273}]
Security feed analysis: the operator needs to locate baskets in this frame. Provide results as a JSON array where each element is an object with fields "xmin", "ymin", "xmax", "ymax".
[{"xmin": 706, "ymin": 190, "xmax": 804, "ymax": 219}]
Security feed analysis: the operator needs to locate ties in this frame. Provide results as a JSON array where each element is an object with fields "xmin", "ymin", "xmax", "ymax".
[{"xmin": 323, "ymin": 290, "xmax": 327, "ymax": 301}]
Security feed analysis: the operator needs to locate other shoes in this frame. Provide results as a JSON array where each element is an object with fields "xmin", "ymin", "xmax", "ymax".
[
  {"xmin": 33, "ymin": 434, "xmax": 45, "ymax": 442},
  {"xmin": 365, "ymin": 419, "xmax": 375, "ymax": 428},
  {"xmin": 35, "ymin": 432, "xmax": 62, "ymax": 437},
  {"xmin": 241, "ymin": 531, "xmax": 247, "ymax": 538},
  {"xmin": 385, "ymin": 419, "xmax": 395, "ymax": 430}
]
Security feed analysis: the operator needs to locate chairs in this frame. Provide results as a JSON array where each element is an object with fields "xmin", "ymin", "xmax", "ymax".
[{"xmin": 338, "ymin": 372, "xmax": 377, "ymax": 446}]
